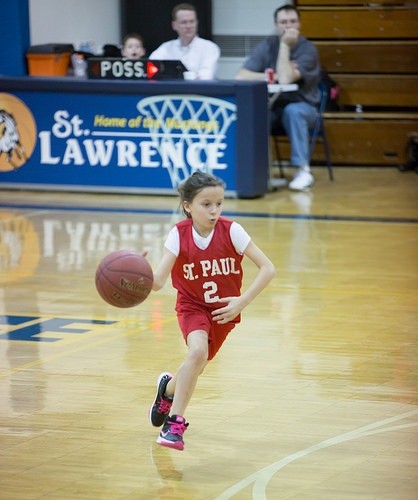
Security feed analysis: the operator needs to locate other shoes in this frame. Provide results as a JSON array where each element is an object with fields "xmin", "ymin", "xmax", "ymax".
[{"xmin": 288, "ymin": 167, "xmax": 314, "ymax": 190}]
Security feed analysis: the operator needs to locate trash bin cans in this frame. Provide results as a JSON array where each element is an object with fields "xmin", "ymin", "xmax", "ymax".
[{"xmin": 26, "ymin": 43, "xmax": 73, "ymax": 76}]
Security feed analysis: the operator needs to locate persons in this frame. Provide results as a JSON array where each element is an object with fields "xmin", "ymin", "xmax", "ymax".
[
  {"xmin": 120, "ymin": 34, "xmax": 145, "ymax": 59},
  {"xmin": 140, "ymin": 173, "xmax": 277, "ymax": 449},
  {"xmin": 234, "ymin": 5, "xmax": 322, "ymax": 191},
  {"xmin": 149, "ymin": 4, "xmax": 221, "ymax": 80}
]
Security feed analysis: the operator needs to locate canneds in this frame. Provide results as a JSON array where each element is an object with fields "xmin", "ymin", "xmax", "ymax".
[{"xmin": 265, "ymin": 68, "xmax": 274, "ymax": 83}]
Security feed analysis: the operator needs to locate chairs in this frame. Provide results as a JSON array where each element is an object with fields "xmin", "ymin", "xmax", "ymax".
[{"xmin": 274, "ymin": 66, "xmax": 337, "ymax": 179}]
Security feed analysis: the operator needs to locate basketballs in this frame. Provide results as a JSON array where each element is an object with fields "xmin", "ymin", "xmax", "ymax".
[{"xmin": 94, "ymin": 249, "xmax": 154, "ymax": 309}]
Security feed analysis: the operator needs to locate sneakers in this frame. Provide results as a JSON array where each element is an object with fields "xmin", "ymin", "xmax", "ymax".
[
  {"xmin": 156, "ymin": 414, "xmax": 189, "ymax": 450},
  {"xmin": 150, "ymin": 371, "xmax": 174, "ymax": 428}
]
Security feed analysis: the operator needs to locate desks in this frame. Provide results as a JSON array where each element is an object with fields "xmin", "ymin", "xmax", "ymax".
[{"xmin": 0, "ymin": 76, "xmax": 271, "ymax": 199}]
